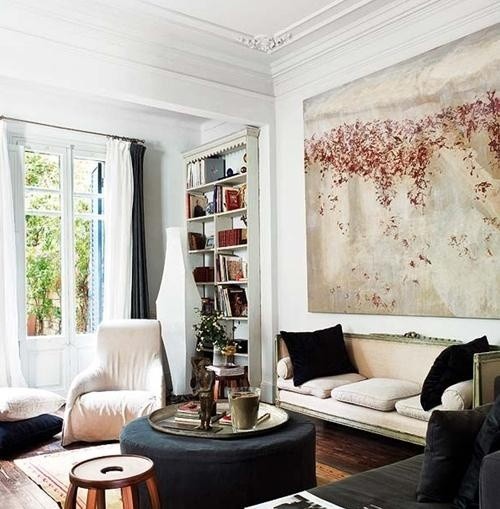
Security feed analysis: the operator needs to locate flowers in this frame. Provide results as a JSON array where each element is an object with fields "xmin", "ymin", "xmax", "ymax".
[{"xmin": 192, "ymin": 304, "xmax": 244, "ymax": 356}]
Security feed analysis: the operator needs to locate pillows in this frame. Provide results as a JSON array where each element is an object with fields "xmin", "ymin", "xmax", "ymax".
[
  {"xmin": 280, "ymin": 324, "xmax": 358, "ymax": 387},
  {"xmin": 417, "ymin": 410, "xmax": 485, "ymax": 503},
  {"xmin": 0, "ymin": 414, "xmax": 62, "ymax": 459},
  {"xmin": 0, "ymin": 387, "xmax": 64, "ymax": 421},
  {"xmin": 420, "ymin": 335, "xmax": 489, "ymax": 411},
  {"xmin": 476, "ymin": 397, "xmax": 500, "ymax": 455}
]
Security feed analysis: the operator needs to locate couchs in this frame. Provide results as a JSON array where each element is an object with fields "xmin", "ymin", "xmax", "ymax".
[
  {"xmin": 60, "ymin": 319, "xmax": 173, "ymax": 448},
  {"xmin": 273, "ymin": 330, "xmax": 500, "ymax": 450},
  {"xmin": 241, "ymin": 450, "xmax": 500, "ymax": 509}
]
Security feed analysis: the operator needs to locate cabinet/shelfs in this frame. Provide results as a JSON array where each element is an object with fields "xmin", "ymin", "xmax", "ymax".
[{"xmin": 181, "ymin": 124, "xmax": 260, "ymax": 398}]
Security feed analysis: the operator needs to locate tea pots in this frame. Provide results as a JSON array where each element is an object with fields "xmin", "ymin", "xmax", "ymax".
[{"xmin": 228, "ymin": 386, "xmax": 262, "ymax": 428}]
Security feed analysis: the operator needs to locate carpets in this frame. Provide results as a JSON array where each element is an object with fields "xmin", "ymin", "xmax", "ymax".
[{"xmin": 13, "ymin": 443, "xmax": 350, "ymax": 509}]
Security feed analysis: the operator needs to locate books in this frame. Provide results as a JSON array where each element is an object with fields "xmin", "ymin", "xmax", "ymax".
[
  {"xmin": 186, "ymin": 155, "xmax": 247, "ymax": 323},
  {"xmin": 175, "ymin": 401, "xmax": 271, "ymax": 428}
]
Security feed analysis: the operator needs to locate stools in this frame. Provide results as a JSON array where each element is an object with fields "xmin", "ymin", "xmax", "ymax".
[{"xmin": 60, "ymin": 453, "xmax": 162, "ymax": 509}]
